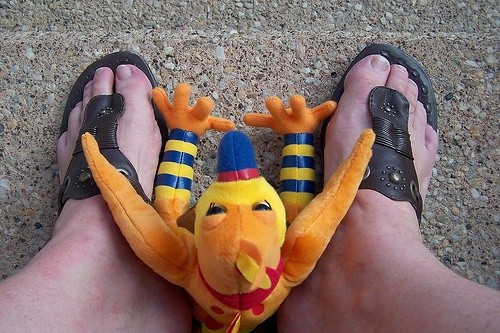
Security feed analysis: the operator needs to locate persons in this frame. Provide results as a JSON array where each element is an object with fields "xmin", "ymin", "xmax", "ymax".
[{"xmin": 0, "ymin": 44, "xmax": 499, "ymax": 332}]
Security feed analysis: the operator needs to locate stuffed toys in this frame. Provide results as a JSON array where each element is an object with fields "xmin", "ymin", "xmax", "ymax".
[{"xmin": 79, "ymin": 81, "xmax": 377, "ymax": 333}]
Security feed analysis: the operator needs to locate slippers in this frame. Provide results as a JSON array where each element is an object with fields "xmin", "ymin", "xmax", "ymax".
[
  {"xmin": 55, "ymin": 47, "xmax": 171, "ymax": 213},
  {"xmin": 316, "ymin": 44, "xmax": 438, "ymax": 227}
]
